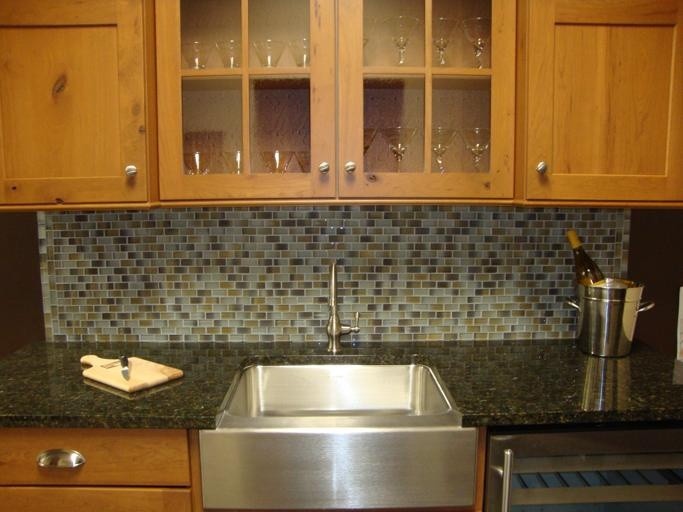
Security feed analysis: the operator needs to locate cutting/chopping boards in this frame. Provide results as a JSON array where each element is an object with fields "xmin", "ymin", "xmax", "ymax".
[{"xmin": 78, "ymin": 354, "xmax": 185, "ymax": 393}]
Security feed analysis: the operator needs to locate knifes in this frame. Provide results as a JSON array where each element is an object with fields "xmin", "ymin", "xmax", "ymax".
[{"xmin": 118, "ymin": 354, "xmax": 130, "ymax": 381}]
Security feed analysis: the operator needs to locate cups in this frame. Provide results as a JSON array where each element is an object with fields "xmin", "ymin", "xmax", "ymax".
[
  {"xmin": 251, "ymin": 41, "xmax": 287, "ymax": 67},
  {"xmin": 181, "ymin": 41, "xmax": 212, "ymax": 69},
  {"xmin": 220, "ymin": 151, "xmax": 253, "ymax": 171},
  {"xmin": 185, "ymin": 152, "xmax": 212, "ymax": 175},
  {"xmin": 290, "ymin": 37, "xmax": 311, "ymax": 66},
  {"xmin": 214, "ymin": 38, "xmax": 252, "ymax": 68},
  {"xmin": 297, "ymin": 152, "xmax": 311, "ymax": 171},
  {"xmin": 258, "ymin": 151, "xmax": 293, "ymax": 173}
]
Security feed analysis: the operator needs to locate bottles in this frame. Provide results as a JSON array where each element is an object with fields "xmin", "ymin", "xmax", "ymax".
[{"xmin": 565, "ymin": 229, "xmax": 607, "ymax": 287}]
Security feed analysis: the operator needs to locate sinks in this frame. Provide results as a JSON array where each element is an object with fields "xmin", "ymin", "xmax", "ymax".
[{"xmin": 218, "ymin": 362, "xmax": 462, "ymax": 427}]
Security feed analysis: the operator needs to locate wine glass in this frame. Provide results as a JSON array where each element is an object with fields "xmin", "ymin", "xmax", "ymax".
[
  {"xmin": 461, "ymin": 15, "xmax": 491, "ymax": 70},
  {"xmin": 460, "ymin": 127, "xmax": 492, "ymax": 173},
  {"xmin": 421, "ymin": 127, "xmax": 459, "ymax": 172},
  {"xmin": 384, "ymin": 14, "xmax": 420, "ymax": 67},
  {"xmin": 425, "ymin": 17, "xmax": 457, "ymax": 67},
  {"xmin": 380, "ymin": 125, "xmax": 417, "ymax": 172}
]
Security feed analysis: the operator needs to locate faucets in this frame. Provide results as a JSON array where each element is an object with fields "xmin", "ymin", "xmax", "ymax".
[{"xmin": 327, "ymin": 260, "xmax": 361, "ymax": 352}]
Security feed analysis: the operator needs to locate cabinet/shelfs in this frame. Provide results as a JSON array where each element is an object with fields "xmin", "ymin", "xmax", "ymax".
[
  {"xmin": 151, "ymin": 0, "xmax": 518, "ymax": 208},
  {"xmin": 0, "ymin": 0, "xmax": 151, "ymax": 213},
  {"xmin": 0, "ymin": 425, "xmax": 204, "ymax": 512},
  {"xmin": 518, "ymin": 0, "xmax": 683, "ymax": 209}
]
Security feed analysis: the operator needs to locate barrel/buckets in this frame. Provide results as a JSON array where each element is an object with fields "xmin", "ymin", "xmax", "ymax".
[
  {"xmin": 566, "ymin": 276, "xmax": 654, "ymax": 356},
  {"xmin": 579, "ymin": 358, "xmax": 632, "ymax": 411}
]
